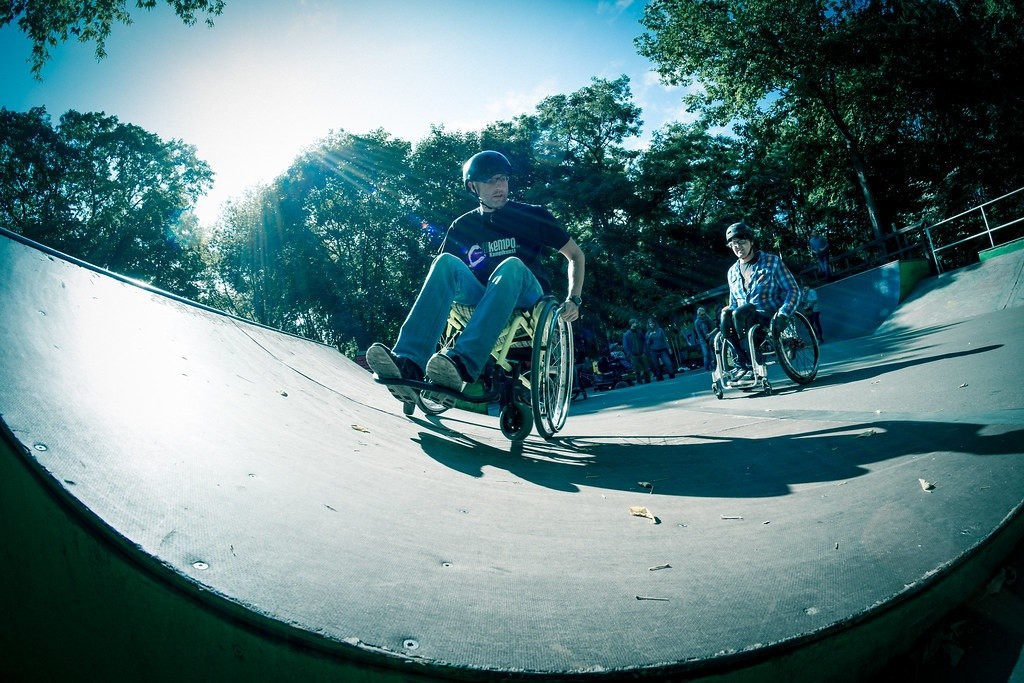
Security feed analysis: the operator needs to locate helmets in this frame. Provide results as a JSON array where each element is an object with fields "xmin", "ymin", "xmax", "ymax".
[
  {"xmin": 725, "ymin": 223, "xmax": 752, "ymax": 242},
  {"xmin": 463, "ymin": 150, "xmax": 511, "ymax": 192}
]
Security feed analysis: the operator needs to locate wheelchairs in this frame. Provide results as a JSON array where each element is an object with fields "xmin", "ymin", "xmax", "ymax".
[
  {"xmin": 372, "ymin": 293, "xmax": 574, "ymax": 441},
  {"xmin": 705, "ymin": 287, "xmax": 820, "ymax": 400}
]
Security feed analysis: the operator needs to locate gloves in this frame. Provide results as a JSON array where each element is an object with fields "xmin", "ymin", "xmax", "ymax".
[{"xmin": 776, "ymin": 315, "xmax": 788, "ymax": 333}]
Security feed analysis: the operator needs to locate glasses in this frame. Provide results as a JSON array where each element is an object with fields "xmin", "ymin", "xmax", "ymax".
[
  {"xmin": 730, "ymin": 239, "xmax": 748, "ymax": 246},
  {"xmin": 486, "ymin": 176, "xmax": 510, "ymax": 184}
]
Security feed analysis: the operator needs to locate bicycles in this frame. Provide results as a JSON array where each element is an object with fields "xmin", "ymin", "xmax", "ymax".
[{"xmin": 518, "ymin": 348, "xmax": 587, "ymax": 408}]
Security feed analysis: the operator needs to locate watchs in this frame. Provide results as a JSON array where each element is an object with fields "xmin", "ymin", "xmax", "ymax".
[{"xmin": 567, "ymin": 293, "xmax": 583, "ymax": 307}]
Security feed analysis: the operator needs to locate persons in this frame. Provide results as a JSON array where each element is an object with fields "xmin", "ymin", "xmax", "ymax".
[
  {"xmin": 363, "ymin": 149, "xmax": 591, "ymax": 405},
  {"xmin": 801, "ymin": 281, "xmax": 825, "ymax": 346},
  {"xmin": 719, "ymin": 222, "xmax": 801, "ymax": 387},
  {"xmin": 623, "ymin": 318, "xmax": 652, "ymax": 385},
  {"xmin": 695, "ymin": 307, "xmax": 717, "ymax": 373},
  {"xmin": 810, "ymin": 229, "xmax": 834, "ymax": 283},
  {"xmin": 645, "ymin": 316, "xmax": 676, "ymax": 381}
]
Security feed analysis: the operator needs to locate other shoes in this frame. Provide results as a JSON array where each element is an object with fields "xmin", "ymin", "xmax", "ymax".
[
  {"xmin": 420, "ymin": 353, "xmax": 463, "ymax": 409},
  {"xmin": 367, "ymin": 343, "xmax": 422, "ymax": 404},
  {"xmin": 738, "ymin": 371, "xmax": 762, "ymax": 385},
  {"xmin": 727, "ymin": 370, "xmax": 746, "ymax": 385}
]
